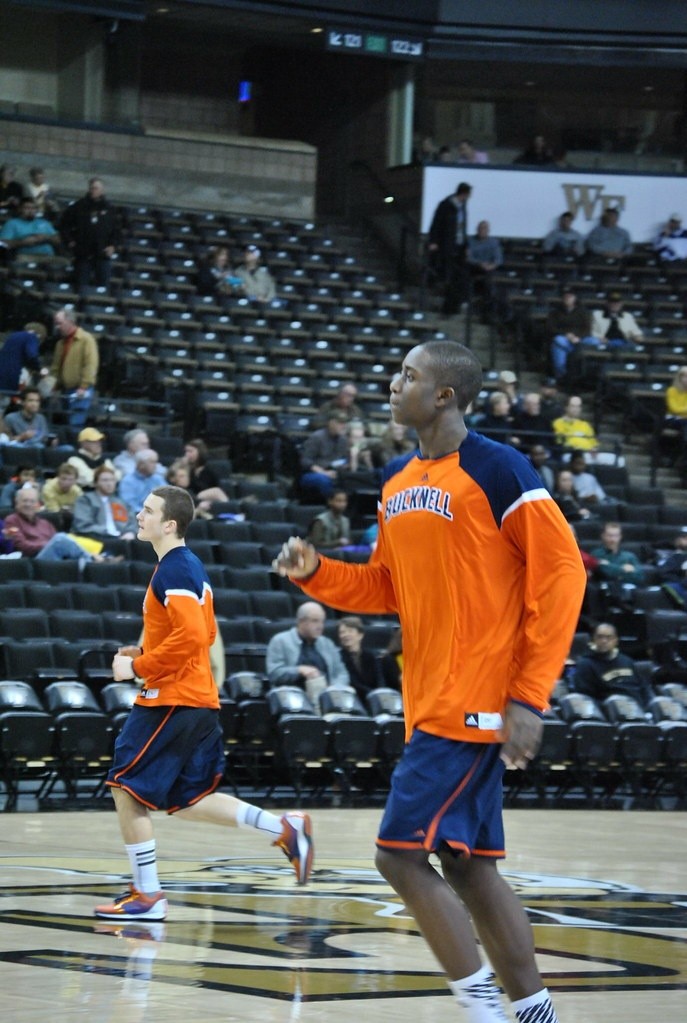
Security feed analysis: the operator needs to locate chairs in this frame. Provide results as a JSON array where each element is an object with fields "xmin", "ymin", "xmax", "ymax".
[
  {"xmin": 0, "ymin": 185, "xmax": 687, "ymax": 810},
  {"xmin": 449, "ymin": 149, "xmax": 683, "ymax": 174}
]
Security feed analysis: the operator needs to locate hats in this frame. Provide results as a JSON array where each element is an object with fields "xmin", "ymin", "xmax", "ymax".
[
  {"xmin": 499, "ymin": 371, "xmax": 517, "ymax": 385},
  {"xmin": 242, "ymin": 245, "xmax": 260, "ymax": 252},
  {"xmin": 677, "ymin": 525, "xmax": 687, "ymax": 536},
  {"xmin": 607, "ymin": 290, "xmax": 623, "ymax": 302},
  {"xmin": 79, "ymin": 428, "xmax": 104, "ymax": 442},
  {"xmin": 325, "ymin": 409, "xmax": 351, "ymax": 423},
  {"xmin": 542, "ymin": 377, "xmax": 557, "ymax": 389}
]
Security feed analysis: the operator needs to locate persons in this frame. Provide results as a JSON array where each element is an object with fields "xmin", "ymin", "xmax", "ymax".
[
  {"xmin": 270, "ymin": 340, "xmax": 588, "ymax": 1023},
  {"xmin": 0, "ymin": 131, "xmax": 687, "ymax": 919}
]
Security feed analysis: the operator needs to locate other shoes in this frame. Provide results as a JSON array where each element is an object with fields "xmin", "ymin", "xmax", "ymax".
[{"xmin": 93, "ymin": 554, "xmax": 126, "ymax": 566}]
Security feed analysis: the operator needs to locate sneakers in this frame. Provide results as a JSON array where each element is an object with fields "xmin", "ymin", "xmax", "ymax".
[
  {"xmin": 272, "ymin": 811, "xmax": 314, "ymax": 885},
  {"xmin": 95, "ymin": 883, "xmax": 167, "ymax": 920},
  {"xmin": 95, "ymin": 921, "xmax": 166, "ymax": 951}
]
